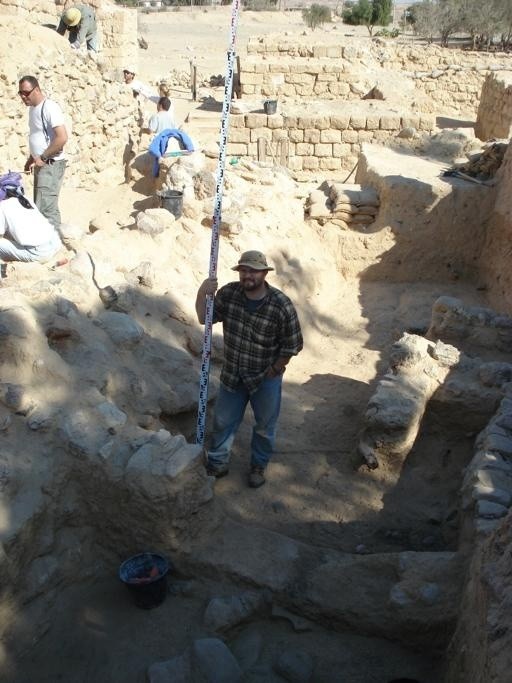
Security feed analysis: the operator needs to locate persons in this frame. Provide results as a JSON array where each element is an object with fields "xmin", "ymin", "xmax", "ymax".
[
  {"xmin": 18, "ymin": 74, "xmax": 71, "ymax": 240},
  {"xmin": 147, "ymin": 95, "xmax": 178, "ymax": 137},
  {"xmin": 140, "ymin": 83, "xmax": 170, "ymax": 102},
  {"xmin": 196, "ymin": 248, "xmax": 304, "ymax": 489},
  {"xmin": 57, "ymin": 3, "xmax": 98, "ymax": 51},
  {"xmin": 121, "ymin": 65, "xmax": 143, "ymax": 91},
  {"xmin": 1, "ymin": 171, "xmax": 64, "ymax": 263}
]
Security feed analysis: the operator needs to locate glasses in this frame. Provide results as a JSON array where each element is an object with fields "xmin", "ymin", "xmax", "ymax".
[{"xmin": 17, "ymin": 84, "xmax": 37, "ymax": 97}]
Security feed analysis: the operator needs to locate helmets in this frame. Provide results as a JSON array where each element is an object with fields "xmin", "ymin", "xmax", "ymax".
[{"xmin": 62, "ymin": 8, "xmax": 82, "ymax": 27}]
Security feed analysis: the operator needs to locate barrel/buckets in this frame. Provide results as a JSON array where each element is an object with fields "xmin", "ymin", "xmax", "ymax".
[
  {"xmin": 263, "ymin": 101, "xmax": 277, "ymax": 115},
  {"xmin": 155, "ymin": 190, "xmax": 183, "ymax": 221},
  {"xmin": 118, "ymin": 552, "xmax": 168, "ymax": 610}
]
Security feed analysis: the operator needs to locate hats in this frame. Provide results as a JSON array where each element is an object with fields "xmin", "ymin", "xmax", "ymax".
[
  {"xmin": 123, "ymin": 68, "xmax": 135, "ymax": 75},
  {"xmin": 231, "ymin": 251, "xmax": 274, "ymax": 271}
]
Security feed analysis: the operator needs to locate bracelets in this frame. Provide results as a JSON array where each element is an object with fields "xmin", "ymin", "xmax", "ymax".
[{"xmin": 40, "ymin": 154, "xmax": 49, "ymax": 162}]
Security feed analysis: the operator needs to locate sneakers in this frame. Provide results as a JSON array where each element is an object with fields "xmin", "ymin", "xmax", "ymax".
[
  {"xmin": 206, "ymin": 466, "xmax": 229, "ymax": 478},
  {"xmin": 248, "ymin": 466, "xmax": 265, "ymax": 489}
]
